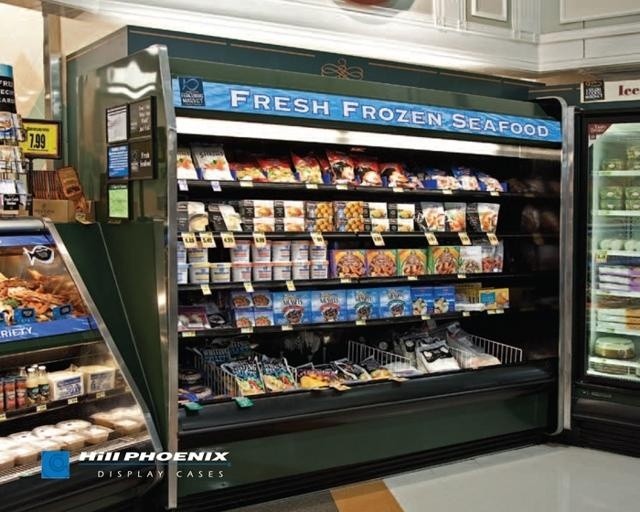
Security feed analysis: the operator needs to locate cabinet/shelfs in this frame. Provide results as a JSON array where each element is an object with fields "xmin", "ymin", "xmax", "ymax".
[
  {"xmin": 0, "ymin": 214, "xmax": 166, "ymax": 512},
  {"xmin": 177, "ymin": 176, "xmax": 556, "ymax": 438}
]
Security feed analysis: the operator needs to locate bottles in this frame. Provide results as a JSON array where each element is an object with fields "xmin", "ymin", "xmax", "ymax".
[{"xmin": 20, "ymin": 363, "xmax": 50, "ymax": 405}]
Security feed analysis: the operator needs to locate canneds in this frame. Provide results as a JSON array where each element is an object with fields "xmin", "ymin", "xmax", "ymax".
[{"xmin": 0, "ymin": 376, "xmax": 27, "ymax": 413}]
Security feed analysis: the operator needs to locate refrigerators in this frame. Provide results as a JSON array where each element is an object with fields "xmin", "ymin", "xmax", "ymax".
[{"xmin": 571, "ymin": 109, "xmax": 640, "ymax": 458}]
